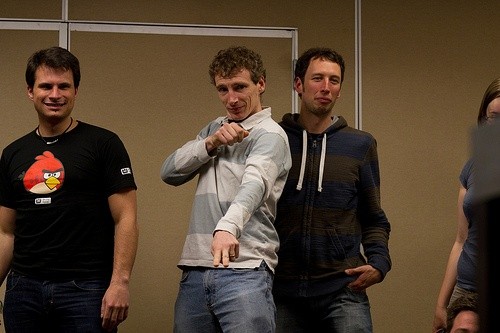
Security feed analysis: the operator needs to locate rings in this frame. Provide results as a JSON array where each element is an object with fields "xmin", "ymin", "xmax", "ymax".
[{"xmin": 230, "ymin": 255, "xmax": 235, "ymax": 257}]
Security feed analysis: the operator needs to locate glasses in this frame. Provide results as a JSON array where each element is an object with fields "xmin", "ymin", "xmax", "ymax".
[{"xmin": 483, "ymin": 114, "xmax": 498, "ymax": 123}]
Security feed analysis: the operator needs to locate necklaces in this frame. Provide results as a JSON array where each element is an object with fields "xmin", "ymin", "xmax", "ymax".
[{"xmin": 38, "ymin": 116, "xmax": 73, "ymax": 144}]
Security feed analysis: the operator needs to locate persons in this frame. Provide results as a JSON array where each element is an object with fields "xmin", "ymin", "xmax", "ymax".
[
  {"xmin": 270, "ymin": 46, "xmax": 392, "ymax": 333},
  {"xmin": 0, "ymin": 46, "xmax": 138, "ymax": 333},
  {"xmin": 160, "ymin": 46, "xmax": 293, "ymax": 333},
  {"xmin": 433, "ymin": 80, "xmax": 500, "ymax": 333}
]
